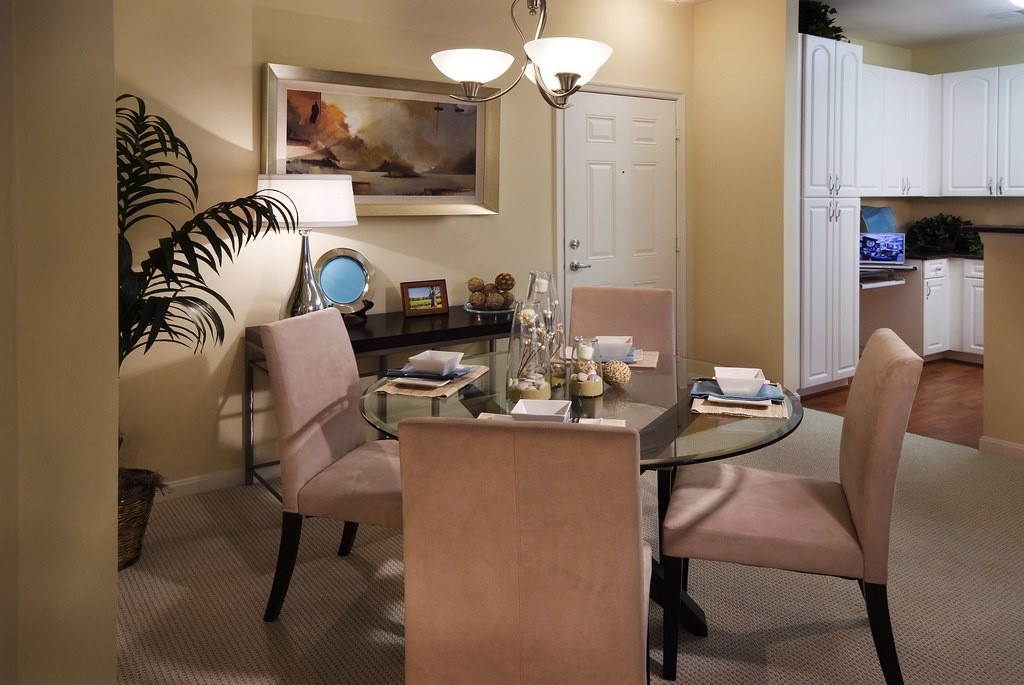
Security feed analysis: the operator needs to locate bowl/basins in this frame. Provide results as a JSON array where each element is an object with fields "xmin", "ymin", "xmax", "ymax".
[
  {"xmin": 407, "ymin": 349, "xmax": 464, "ymax": 376},
  {"xmin": 595, "ymin": 334, "xmax": 633, "ymax": 358},
  {"xmin": 511, "ymin": 398, "xmax": 571, "ymax": 423},
  {"xmin": 713, "ymin": 365, "xmax": 765, "ymax": 398}
]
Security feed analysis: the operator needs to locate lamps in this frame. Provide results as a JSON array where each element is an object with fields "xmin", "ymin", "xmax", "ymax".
[
  {"xmin": 430, "ymin": 1, "xmax": 615, "ymax": 109},
  {"xmin": 254, "ymin": 172, "xmax": 359, "ymax": 317}
]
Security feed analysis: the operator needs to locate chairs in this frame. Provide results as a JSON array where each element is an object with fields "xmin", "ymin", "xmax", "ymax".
[
  {"xmin": 659, "ymin": 326, "xmax": 924, "ymax": 685},
  {"xmin": 567, "ymin": 287, "xmax": 674, "ymax": 358},
  {"xmin": 397, "ymin": 415, "xmax": 651, "ymax": 685},
  {"xmin": 259, "ymin": 306, "xmax": 401, "ymax": 623}
]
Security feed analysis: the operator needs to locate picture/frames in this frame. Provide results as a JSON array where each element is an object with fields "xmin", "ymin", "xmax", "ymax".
[
  {"xmin": 263, "ymin": 62, "xmax": 500, "ymax": 224},
  {"xmin": 399, "ymin": 279, "xmax": 449, "ymax": 316}
]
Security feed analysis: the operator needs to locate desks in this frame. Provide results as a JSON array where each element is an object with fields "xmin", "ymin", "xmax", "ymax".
[
  {"xmin": 358, "ymin": 344, "xmax": 804, "ymax": 468},
  {"xmin": 245, "ymin": 305, "xmax": 521, "ymax": 503}
]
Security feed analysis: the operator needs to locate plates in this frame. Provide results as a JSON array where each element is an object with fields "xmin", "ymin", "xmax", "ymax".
[
  {"xmin": 564, "ymin": 345, "xmax": 643, "ymax": 362},
  {"xmin": 392, "ymin": 364, "xmax": 465, "ymax": 387},
  {"xmin": 491, "ymin": 413, "xmax": 603, "ymax": 425},
  {"xmin": 708, "ymin": 376, "xmax": 771, "ymax": 406},
  {"xmin": 464, "ymin": 302, "xmax": 523, "ymax": 314}
]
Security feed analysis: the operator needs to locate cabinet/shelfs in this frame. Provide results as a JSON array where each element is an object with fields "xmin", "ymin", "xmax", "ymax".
[
  {"xmin": 915, "ymin": 256, "xmax": 985, "ymax": 356},
  {"xmin": 798, "ymin": 31, "xmax": 862, "ymax": 397},
  {"xmin": 861, "ymin": 63, "xmax": 943, "ymax": 199},
  {"xmin": 940, "ymin": 62, "xmax": 1024, "ymax": 197}
]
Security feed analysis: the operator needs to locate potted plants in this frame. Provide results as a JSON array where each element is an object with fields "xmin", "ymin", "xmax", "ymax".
[{"xmin": 116, "ymin": 95, "xmax": 301, "ymax": 569}]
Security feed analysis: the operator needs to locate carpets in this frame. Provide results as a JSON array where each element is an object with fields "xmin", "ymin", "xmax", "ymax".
[{"xmin": 115, "ymin": 410, "xmax": 1024, "ymax": 685}]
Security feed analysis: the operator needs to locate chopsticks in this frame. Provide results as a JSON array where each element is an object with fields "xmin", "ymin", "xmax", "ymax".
[
  {"xmin": 572, "ymin": 415, "xmax": 581, "ymax": 423},
  {"xmin": 386, "ymin": 370, "xmax": 458, "ymax": 381}
]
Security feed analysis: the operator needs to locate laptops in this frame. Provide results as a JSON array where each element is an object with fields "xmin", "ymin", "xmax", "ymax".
[{"xmin": 860, "ymin": 232, "xmax": 914, "ymax": 270}]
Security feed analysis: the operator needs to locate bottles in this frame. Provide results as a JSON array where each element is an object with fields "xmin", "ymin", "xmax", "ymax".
[
  {"xmin": 505, "ymin": 302, "xmax": 552, "ymax": 399},
  {"xmin": 568, "ymin": 335, "xmax": 604, "ymax": 397},
  {"xmin": 526, "ymin": 270, "xmax": 567, "ymax": 388}
]
